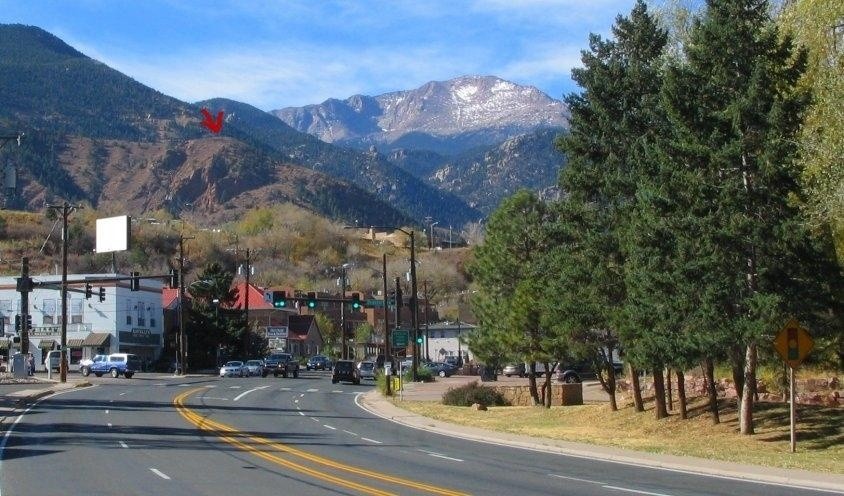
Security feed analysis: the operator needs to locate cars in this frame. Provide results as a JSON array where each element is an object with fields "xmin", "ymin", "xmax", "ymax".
[
  {"xmin": 555, "ymin": 347, "xmax": 622, "ymax": 383},
  {"xmin": 220, "ymin": 360, "xmax": 262, "ymax": 378},
  {"xmin": 332, "ymin": 360, "xmax": 360, "ymax": 385},
  {"xmin": 503, "ymin": 362, "xmax": 525, "ymax": 377},
  {"xmin": 307, "ymin": 355, "xmax": 332, "ymax": 371},
  {"xmin": 424, "ymin": 362, "xmax": 459, "ymax": 378}
]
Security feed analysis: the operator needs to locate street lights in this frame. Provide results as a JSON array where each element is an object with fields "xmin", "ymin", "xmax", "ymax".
[
  {"xmin": 238, "ymin": 248, "xmax": 255, "ymax": 326},
  {"xmin": 417, "ymin": 280, "xmax": 437, "ymax": 359},
  {"xmin": 332, "ymin": 226, "xmax": 417, "ymax": 384},
  {"xmin": 430, "ymin": 221, "xmax": 440, "ymax": 249},
  {"xmin": 295, "ymin": 300, "xmax": 301, "ymax": 315},
  {"xmin": 446, "ymin": 320, "xmax": 460, "ymax": 360}
]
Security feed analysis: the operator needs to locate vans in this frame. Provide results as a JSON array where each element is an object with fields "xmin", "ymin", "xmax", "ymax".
[
  {"xmin": 79, "ymin": 353, "xmax": 140, "ymax": 378},
  {"xmin": 45, "ymin": 350, "xmax": 61, "ymax": 373}
]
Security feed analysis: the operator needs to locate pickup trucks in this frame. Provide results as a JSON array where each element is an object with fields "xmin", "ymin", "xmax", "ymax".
[{"xmin": 262, "ymin": 353, "xmax": 300, "ymax": 378}]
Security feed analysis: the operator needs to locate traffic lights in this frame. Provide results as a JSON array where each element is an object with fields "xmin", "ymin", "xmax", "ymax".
[
  {"xmin": 14, "ymin": 315, "xmax": 33, "ymax": 333},
  {"xmin": 86, "ymin": 283, "xmax": 92, "ymax": 300},
  {"xmin": 171, "ymin": 269, "xmax": 179, "ymax": 289},
  {"xmin": 417, "ymin": 337, "xmax": 423, "ymax": 343},
  {"xmin": 308, "ymin": 292, "xmax": 315, "ymax": 308},
  {"xmin": 131, "ymin": 271, "xmax": 139, "ymax": 291},
  {"xmin": 273, "ymin": 291, "xmax": 287, "ymax": 307},
  {"xmin": 100, "ymin": 286, "xmax": 105, "ymax": 303}
]
